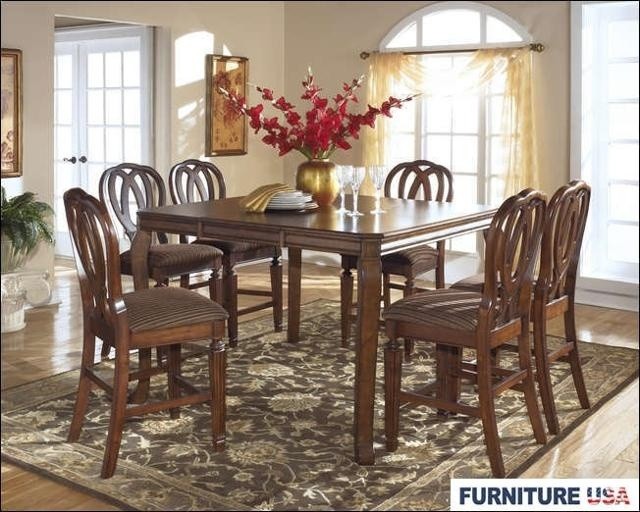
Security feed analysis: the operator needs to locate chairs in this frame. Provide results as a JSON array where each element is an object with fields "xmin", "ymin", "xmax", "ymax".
[
  {"xmin": 63, "ymin": 187, "xmax": 233, "ymax": 479},
  {"xmin": 447, "ymin": 180, "xmax": 594, "ymax": 437},
  {"xmin": 97, "ymin": 162, "xmax": 227, "ymax": 371},
  {"xmin": 377, "ymin": 186, "xmax": 553, "ymax": 478},
  {"xmin": 338, "ymin": 159, "xmax": 455, "ymax": 357},
  {"xmin": 168, "ymin": 159, "xmax": 285, "ymax": 349}
]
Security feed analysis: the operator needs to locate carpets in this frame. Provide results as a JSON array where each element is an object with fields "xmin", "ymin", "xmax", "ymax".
[{"xmin": 0, "ymin": 296, "xmax": 640, "ymax": 510}]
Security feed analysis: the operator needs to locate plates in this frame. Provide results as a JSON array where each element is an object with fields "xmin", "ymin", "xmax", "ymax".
[{"xmin": 265, "ymin": 189, "xmax": 320, "ymax": 210}]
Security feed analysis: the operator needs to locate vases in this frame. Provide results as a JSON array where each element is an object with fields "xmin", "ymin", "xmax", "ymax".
[{"xmin": 294, "ymin": 159, "xmax": 341, "ymax": 207}]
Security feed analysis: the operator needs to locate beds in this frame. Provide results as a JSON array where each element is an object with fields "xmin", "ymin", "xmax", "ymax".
[{"xmin": 133, "ymin": 192, "xmax": 514, "ymax": 468}]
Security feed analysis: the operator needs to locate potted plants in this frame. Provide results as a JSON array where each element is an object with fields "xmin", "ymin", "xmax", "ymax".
[{"xmin": 1, "ymin": 186, "xmax": 58, "ymax": 274}]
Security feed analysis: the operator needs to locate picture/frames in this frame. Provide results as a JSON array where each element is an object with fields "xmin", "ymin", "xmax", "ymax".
[
  {"xmin": 1, "ymin": 47, "xmax": 26, "ymax": 179},
  {"xmin": 205, "ymin": 54, "xmax": 250, "ymax": 158}
]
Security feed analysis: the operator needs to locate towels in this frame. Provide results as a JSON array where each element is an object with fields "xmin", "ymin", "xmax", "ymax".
[{"xmin": 238, "ymin": 183, "xmax": 294, "ymax": 214}]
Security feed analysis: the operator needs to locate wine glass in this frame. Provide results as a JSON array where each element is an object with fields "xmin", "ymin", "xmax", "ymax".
[
  {"xmin": 369, "ymin": 165, "xmax": 388, "ymax": 214},
  {"xmin": 334, "ymin": 164, "xmax": 353, "ymax": 213},
  {"xmin": 346, "ymin": 167, "xmax": 367, "ymax": 216}
]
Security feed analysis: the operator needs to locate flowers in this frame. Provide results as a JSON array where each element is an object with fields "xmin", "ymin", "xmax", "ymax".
[{"xmin": 222, "ymin": 68, "xmax": 425, "ymax": 164}]
